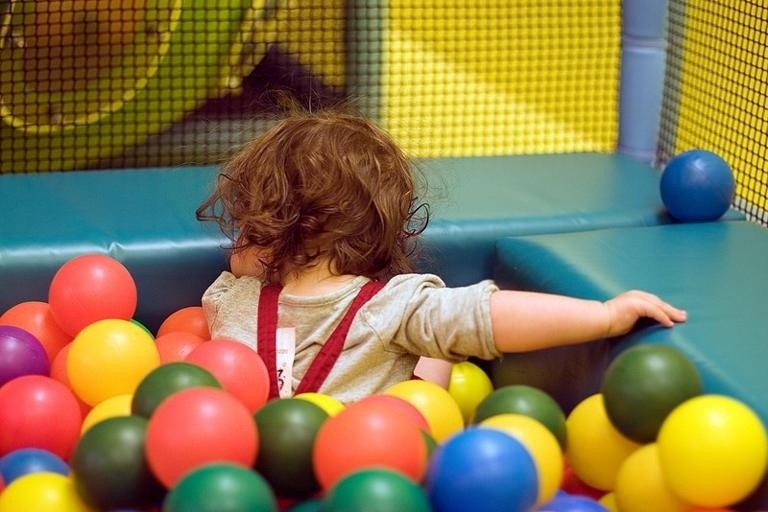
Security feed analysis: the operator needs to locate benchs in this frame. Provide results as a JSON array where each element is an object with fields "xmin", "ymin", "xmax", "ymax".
[
  {"xmin": 0, "ymin": 149, "xmax": 748, "ymax": 329},
  {"xmin": 491, "ymin": 218, "xmax": 768, "ymax": 457}
]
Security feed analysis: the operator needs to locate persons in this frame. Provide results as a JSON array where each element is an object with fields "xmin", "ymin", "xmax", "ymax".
[{"xmin": 194, "ymin": 88, "xmax": 687, "ymax": 402}]
